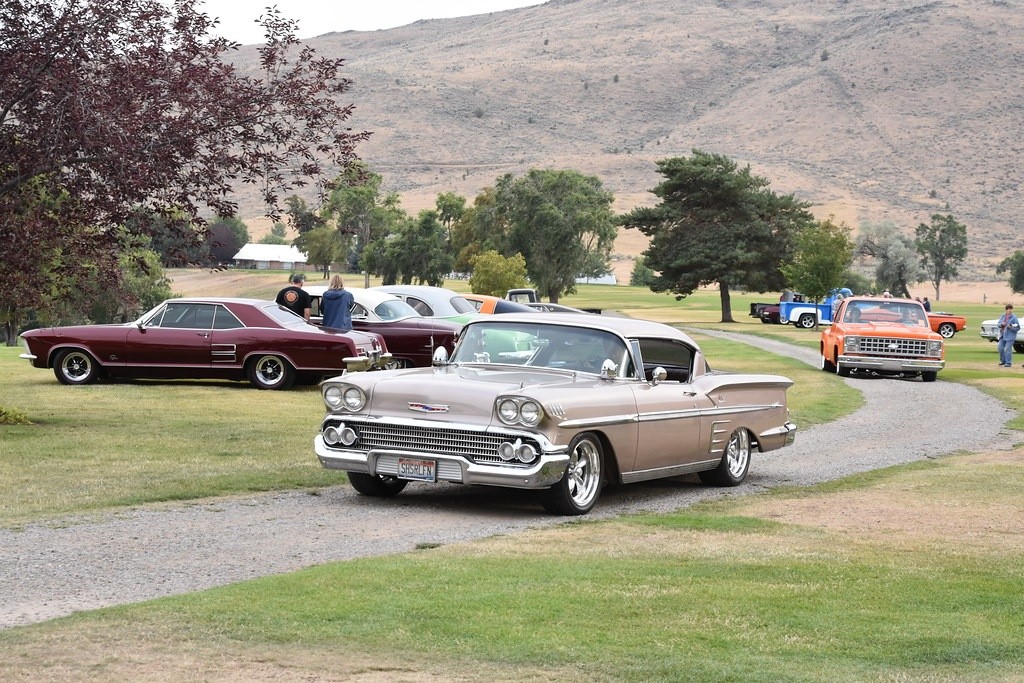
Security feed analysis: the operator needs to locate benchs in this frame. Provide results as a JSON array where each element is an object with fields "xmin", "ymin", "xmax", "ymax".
[{"xmin": 645, "ymin": 369, "xmax": 689, "ymax": 383}]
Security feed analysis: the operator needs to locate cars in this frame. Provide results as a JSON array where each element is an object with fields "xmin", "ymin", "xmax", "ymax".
[
  {"xmin": 843, "ymin": 304, "xmax": 968, "ymax": 340},
  {"xmin": 282, "ymin": 284, "xmax": 633, "ymax": 373},
  {"xmin": 979, "ymin": 317, "xmax": 1024, "ymax": 354},
  {"xmin": 821, "ymin": 296, "xmax": 946, "ymax": 382},
  {"xmin": 18, "ymin": 297, "xmax": 390, "ymax": 392},
  {"xmin": 314, "ymin": 310, "xmax": 799, "ymax": 517}
]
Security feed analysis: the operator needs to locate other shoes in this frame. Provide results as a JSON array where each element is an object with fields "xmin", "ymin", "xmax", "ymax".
[
  {"xmin": 999, "ymin": 362, "xmax": 1005, "ymax": 365},
  {"xmin": 1004, "ymin": 364, "xmax": 1010, "ymax": 367}
]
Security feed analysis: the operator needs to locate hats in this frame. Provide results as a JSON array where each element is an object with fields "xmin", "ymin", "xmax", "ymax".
[{"xmin": 293, "ymin": 273, "xmax": 305, "ymax": 282}]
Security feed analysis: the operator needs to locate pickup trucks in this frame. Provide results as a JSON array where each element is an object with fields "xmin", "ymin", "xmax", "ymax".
[
  {"xmin": 749, "ymin": 293, "xmax": 814, "ymax": 325},
  {"xmin": 778, "ymin": 288, "xmax": 872, "ymax": 329}
]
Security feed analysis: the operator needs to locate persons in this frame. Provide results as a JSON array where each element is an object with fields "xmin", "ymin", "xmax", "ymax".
[
  {"xmin": 598, "ymin": 340, "xmax": 623, "ymax": 370},
  {"xmin": 320, "ymin": 275, "xmax": 354, "ymax": 330},
  {"xmin": 897, "ymin": 310, "xmax": 917, "ymax": 324},
  {"xmin": 793, "ymin": 297, "xmax": 800, "ymax": 302},
  {"xmin": 996, "ymin": 305, "xmax": 1020, "ymax": 368},
  {"xmin": 276, "ymin": 274, "xmax": 311, "ymax": 321},
  {"xmin": 849, "ymin": 309, "xmax": 861, "ymax": 322},
  {"xmin": 834, "ymin": 290, "xmax": 931, "ymax": 312}
]
